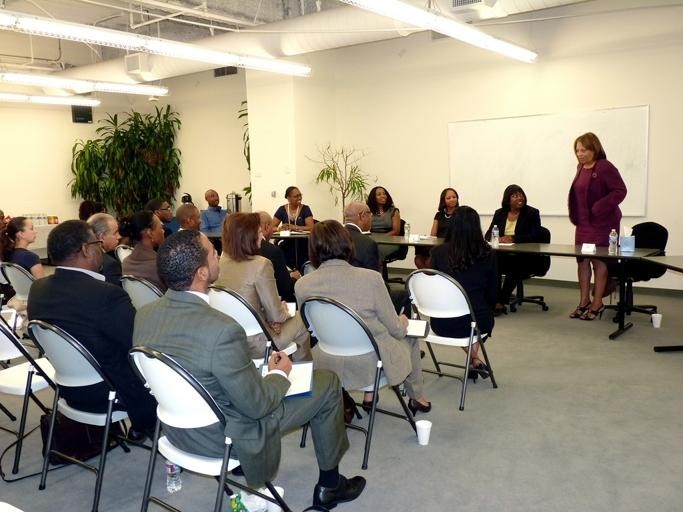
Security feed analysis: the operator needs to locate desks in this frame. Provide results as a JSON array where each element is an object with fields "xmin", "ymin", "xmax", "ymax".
[
  {"xmin": 641, "ymin": 257, "xmax": 683, "ymax": 353},
  {"xmin": 204, "ymin": 230, "xmax": 659, "ymax": 340}
]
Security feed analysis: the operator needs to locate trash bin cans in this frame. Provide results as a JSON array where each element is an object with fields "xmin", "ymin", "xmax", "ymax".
[{"xmin": 227, "ymin": 191, "xmax": 242, "ymax": 215}]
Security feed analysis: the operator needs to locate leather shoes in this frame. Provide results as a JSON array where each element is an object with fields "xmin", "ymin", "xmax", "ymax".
[
  {"xmin": 312, "ymin": 474, "xmax": 366, "ymax": 511},
  {"xmin": 126, "ymin": 427, "xmax": 146, "ymax": 446}
]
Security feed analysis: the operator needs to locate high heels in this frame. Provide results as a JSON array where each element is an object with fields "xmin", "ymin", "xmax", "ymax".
[
  {"xmin": 471, "ymin": 357, "xmax": 493, "ymax": 379},
  {"xmin": 362, "ymin": 393, "xmax": 379, "ymax": 415},
  {"xmin": 408, "ymin": 398, "xmax": 431, "ymax": 417},
  {"xmin": 569, "ymin": 301, "xmax": 591, "ymax": 318},
  {"xmin": 580, "ymin": 302, "xmax": 606, "ymax": 320},
  {"xmin": 22, "ymin": 333, "xmax": 29, "ymax": 340},
  {"xmin": 468, "ymin": 369, "xmax": 478, "ymax": 383}
]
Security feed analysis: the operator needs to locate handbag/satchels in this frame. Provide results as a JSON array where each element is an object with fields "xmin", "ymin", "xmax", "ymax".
[{"xmin": 0, "ymin": 408, "xmax": 128, "ymax": 482}]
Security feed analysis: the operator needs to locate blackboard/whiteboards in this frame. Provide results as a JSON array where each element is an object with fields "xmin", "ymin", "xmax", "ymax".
[{"xmin": 446, "ymin": 103, "xmax": 651, "ymax": 218}]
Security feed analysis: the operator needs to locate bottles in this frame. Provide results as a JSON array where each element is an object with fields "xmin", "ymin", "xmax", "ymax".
[
  {"xmin": 165, "ymin": 460, "xmax": 182, "ymax": 494},
  {"xmin": 23, "ymin": 213, "xmax": 47, "ymax": 225},
  {"xmin": 404, "ymin": 222, "xmax": 411, "ymax": 239},
  {"xmin": 608, "ymin": 229, "xmax": 618, "ymax": 254},
  {"xmin": 491, "ymin": 225, "xmax": 499, "ymax": 249}
]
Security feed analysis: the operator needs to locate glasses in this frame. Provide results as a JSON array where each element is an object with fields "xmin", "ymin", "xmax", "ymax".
[
  {"xmin": 77, "ymin": 239, "xmax": 104, "ymax": 253},
  {"xmin": 153, "ymin": 207, "xmax": 170, "ymax": 211}
]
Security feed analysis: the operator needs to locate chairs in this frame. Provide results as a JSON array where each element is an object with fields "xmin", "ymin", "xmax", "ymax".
[
  {"xmin": 0, "ymin": 314, "xmax": 21, "ymax": 340},
  {"xmin": 0, "ymin": 264, "xmax": 36, "ymax": 335},
  {"xmin": 301, "ymin": 261, "xmax": 315, "ymax": 277},
  {"xmin": 592, "ymin": 222, "xmax": 669, "ymax": 323},
  {"xmin": 205, "ymin": 285, "xmax": 297, "ymax": 369},
  {"xmin": 0, "ymin": 324, "xmax": 57, "ymax": 474},
  {"xmin": 114, "ymin": 245, "xmax": 133, "ymax": 264},
  {"xmin": 274, "ymin": 219, "xmax": 319, "ymax": 246},
  {"xmin": 300, "ymin": 296, "xmax": 418, "ymax": 470},
  {"xmin": 0, "ymin": 266, "xmax": 10, "ymax": 307},
  {"xmin": 502, "ymin": 227, "xmax": 551, "ymax": 312},
  {"xmin": 119, "ymin": 275, "xmax": 164, "ymax": 311},
  {"xmin": 128, "ymin": 346, "xmax": 293, "ymax": 511},
  {"xmin": 402, "ymin": 269, "xmax": 498, "ymax": 411},
  {"xmin": 381, "ymin": 218, "xmax": 409, "ymax": 290},
  {"xmin": 27, "ymin": 319, "xmax": 184, "ymax": 511}
]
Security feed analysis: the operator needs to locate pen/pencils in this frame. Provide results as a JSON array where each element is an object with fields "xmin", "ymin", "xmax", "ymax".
[{"xmin": 399, "ymin": 307, "xmax": 405, "ymax": 316}]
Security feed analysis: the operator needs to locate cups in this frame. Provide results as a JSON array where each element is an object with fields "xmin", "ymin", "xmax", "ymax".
[
  {"xmin": 416, "ymin": 420, "xmax": 432, "ymax": 445},
  {"xmin": 414, "ymin": 234, "xmax": 419, "ymax": 241},
  {"xmin": 265, "ymin": 486, "xmax": 284, "ymax": 512},
  {"xmin": 409, "ymin": 234, "xmax": 413, "ymax": 242},
  {"xmin": 652, "ymin": 313, "xmax": 662, "ymax": 329}
]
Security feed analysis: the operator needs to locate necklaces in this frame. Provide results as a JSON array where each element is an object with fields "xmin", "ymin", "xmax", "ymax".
[{"xmin": 445, "ymin": 213, "xmax": 453, "ymax": 219}]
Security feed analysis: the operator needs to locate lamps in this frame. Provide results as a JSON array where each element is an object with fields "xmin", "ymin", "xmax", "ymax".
[
  {"xmin": 0, "ymin": 10, "xmax": 312, "ymax": 79},
  {"xmin": 0, "ymin": 72, "xmax": 168, "ymax": 96},
  {"xmin": 341, "ymin": 1, "xmax": 538, "ymax": 65},
  {"xmin": 0, "ymin": 92, "xmax": 101, "ymax": 107}
]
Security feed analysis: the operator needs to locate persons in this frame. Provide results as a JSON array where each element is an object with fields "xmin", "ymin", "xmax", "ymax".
[
  {"xmin": 213, "ymin": 213, "xmax": 313, "ymax": 362},
  {"xmin": 122, "ymin": 209, "xmax": 167, "ymax": 297},
  {"xmin": 145, "ymin": 198, "xmax": 174, "ymax": 239},
  {"xmin": 366, "ymin": 185, "xmax": 401, "ymax": 265},
  {"xmin": 294, "ymin": 220, "xmax": 432, "ymax": 418},
  {"xmin": 567, "ymin": 131, "xmax": 628, "ymax": 321},
  {"xmin": 132, "ymin": 228, "xmax": 366, "ymax": 512},
  {"xmin": 27, "ymin": 219, "xmax": 161, "ymax": 444},
  {"xmin": 0, "ymin": 216, "xmax": 44, "ymax": 280},
  {"xmin": 0, "ymin": 208, "xmax": 7, "ymax": 231},
  {"xmin": 485, "ymin": 183, "xmax": 542, "ymax": 318},
  {"xmin": 199, "ymin": 189, "xmax": 230, "ymax": 235},
  {"xmin": 270, "ymin": 185, "xmax": 315, "ymax": 249},
  {"xmin": 260, "ymin": 211, "xmax": 303, "ymax": 304},
  {"xmin": 176, "ymin": 204, "xmax": 204, "ymax": 231},
  {"xmin": 342, "ymin": 201, "xmax": 380, "ymax": 273},
  {"xmin": 430, "ymin": 205, "xmax": 499, "ymax": 385},
  {"xmin": 414, "ymin": 187, "xmax": 461, "ymax": 271}
]
